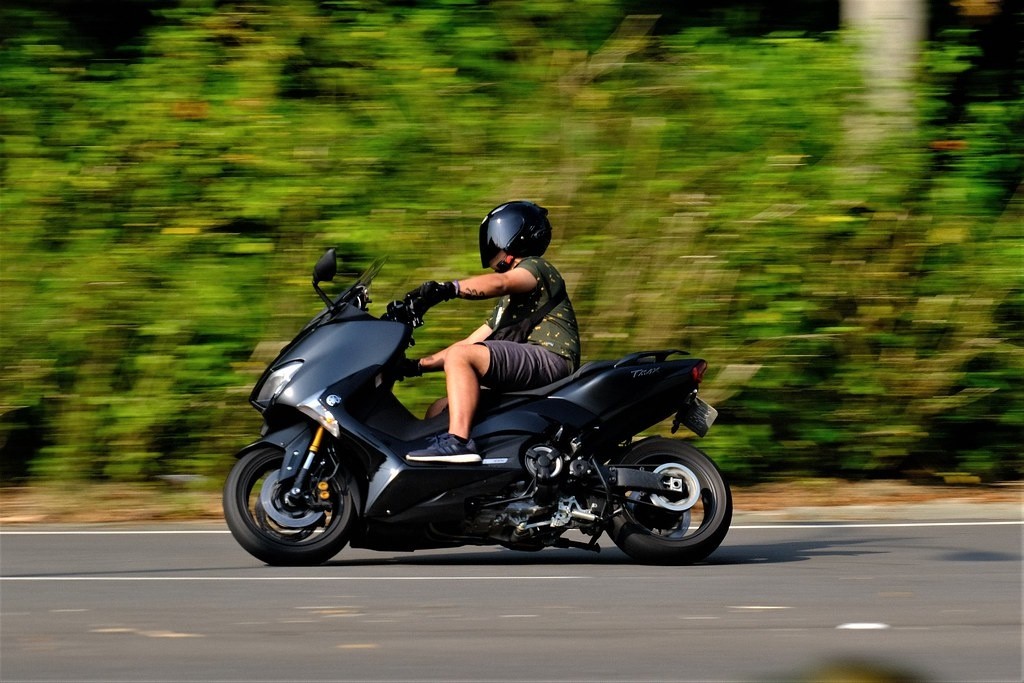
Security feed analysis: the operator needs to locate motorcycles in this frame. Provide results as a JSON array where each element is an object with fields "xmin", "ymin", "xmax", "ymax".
[{"xmin": 222, "ymin": 247, "xmax": 733, "ymax": 566}]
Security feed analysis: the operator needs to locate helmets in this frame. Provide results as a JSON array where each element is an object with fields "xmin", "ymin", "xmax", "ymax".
[{"xmin": 479, "ymin": 200, "xmax": 552, "ymax": 269}]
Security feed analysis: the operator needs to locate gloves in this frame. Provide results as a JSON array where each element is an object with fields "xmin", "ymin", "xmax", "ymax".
[{"xmin": 405, "ymin": 280, "xmax": 457, "ymax": 315}]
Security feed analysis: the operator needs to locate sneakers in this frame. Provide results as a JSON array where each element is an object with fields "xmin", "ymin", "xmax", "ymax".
[{"xmin": 405, "ymin": 433, "xmax": 482, "ymax": 463}]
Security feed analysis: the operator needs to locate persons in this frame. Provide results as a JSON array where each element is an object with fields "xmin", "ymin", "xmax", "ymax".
[{"xmin": 405, "ymin": 201, "xmax": 582, "ymax": 463}]
